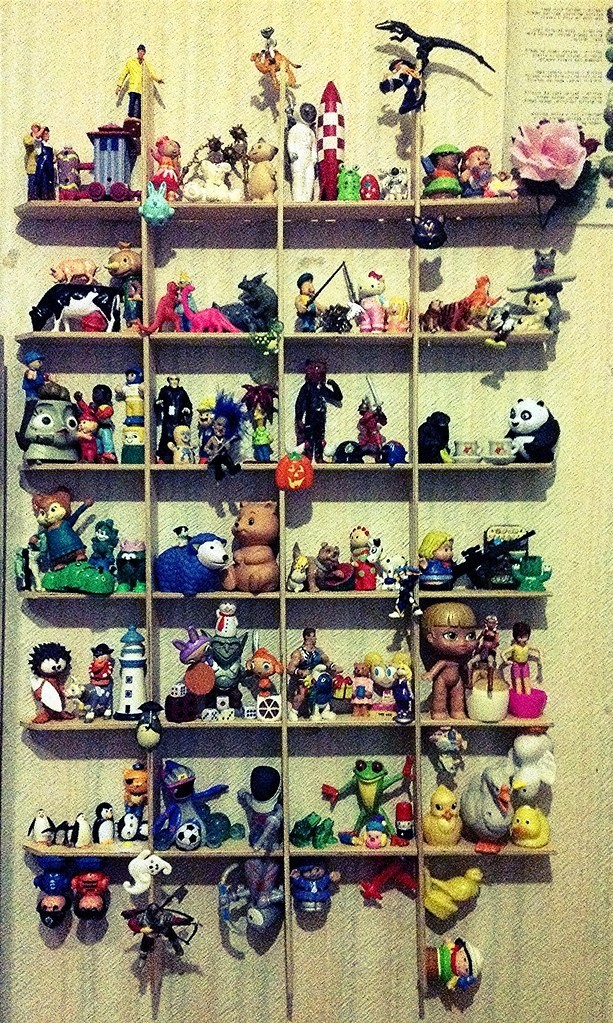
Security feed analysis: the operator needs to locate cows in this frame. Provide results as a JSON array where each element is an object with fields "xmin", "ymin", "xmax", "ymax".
[
  {"xmin": 29, "ymin": 284, "xmax": 120, "ymax": 331},
  {"xmin": 49, "ymin": 262, "xmax": 105, "ymax": 284}
]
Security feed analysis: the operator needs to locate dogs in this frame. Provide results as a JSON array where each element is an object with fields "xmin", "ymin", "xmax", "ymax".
[{"xmin": 293, "ymin": 541, "xmax": 344, "ymax": 593}]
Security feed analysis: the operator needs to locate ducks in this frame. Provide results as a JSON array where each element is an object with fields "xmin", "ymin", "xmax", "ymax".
[
  {"xmin": 512, "ymin": 803, "xmax": 551, "ymax": 848},
  {"xmin": 422, "ymin": 783, "xmax": 462, "ymax": 847}
]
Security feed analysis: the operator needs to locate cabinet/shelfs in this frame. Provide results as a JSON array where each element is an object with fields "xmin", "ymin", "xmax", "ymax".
[{"xmin": 15, "ymin": 72, "xmax": 558, "ymax": 1004}]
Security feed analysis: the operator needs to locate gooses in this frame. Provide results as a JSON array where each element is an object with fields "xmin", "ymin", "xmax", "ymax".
[
  {"xmin": 507, "ymin": 727, "xmax": 556, "ymax": 800},
  {"xmin": 462, "ymin": 765, "xmax": 514, "ymax": 857}
]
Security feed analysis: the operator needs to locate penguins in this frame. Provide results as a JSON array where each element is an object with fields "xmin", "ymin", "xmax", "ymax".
[{"xmin": 26, "ymin": 802, "xmax": 149, "ymax": 848}]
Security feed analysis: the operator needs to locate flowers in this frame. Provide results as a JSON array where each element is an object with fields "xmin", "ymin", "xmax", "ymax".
[{"xmin": 509, "ymin": 117, "xmax": 602, "ymax": 206}]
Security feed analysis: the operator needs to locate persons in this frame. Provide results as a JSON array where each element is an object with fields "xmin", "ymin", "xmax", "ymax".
[
  {"xmin": 421, "ymin": 146, "xmax": 523, "ymax": 199},
  {"xmin": 156, "ymin": 375, "xmax": 278, "ymax": 481},
  {"xmin": 386, "ymin": 300, "xmax": 410, "ymax": 336},
  {"xmin": 237, "ymin": 766, "xmax": 283, "ymax": 851},
  {"xmin": 260, "ymin": 28, "xmax": 277, "ymax": 64},
  {"xmin": 287, "ymin": 103, "xmax": 319, "ymax": 203},
  {"xmin": 421, "ymin": 604, "xmax": 541, "ymax": 720},
  {"xmin": 33, "ymin": 858, "xmax": 71, "ymax": 928},
  {"xmin": 288, "ymin": 629, "xmax": 343, "ymax": 720},
  {"xmin": 115, "ymin": 45, "xmax": 164, "ymax": 118},
  {"xmin": 148, "ymin": 138, "xmax": 182, "ymax": 200},
  {"xmin": 25, "ymin": 122, "xmax": 56, "ymax": 201},
  {"xmin": 291, "ymin": 864, "xmax": 341, "ymax": 912},
  {"xmin": 71, "ymin": 858, "xmax": 110, "ymax": 920},
  {"xmin": 17, "ymin": 352, "xmax": 143, "ymax": 462},
  {"xmin": 425, "ymin": 937, "xmax": 482, "ymax": 991},
  {"xmin": 419, "ymin": 532, "xmax": 454, "ymax": 590},
  {"xmin": 294, "ymin": 358, "xmax": 384, "ymax": 463},
  {"xmin": 384, "ymin": 168, "xmax": 404, "ymax": 201},
  {"xmin": 295, "ymin": 273, "xmax": 326, "ymax": 331}
]
